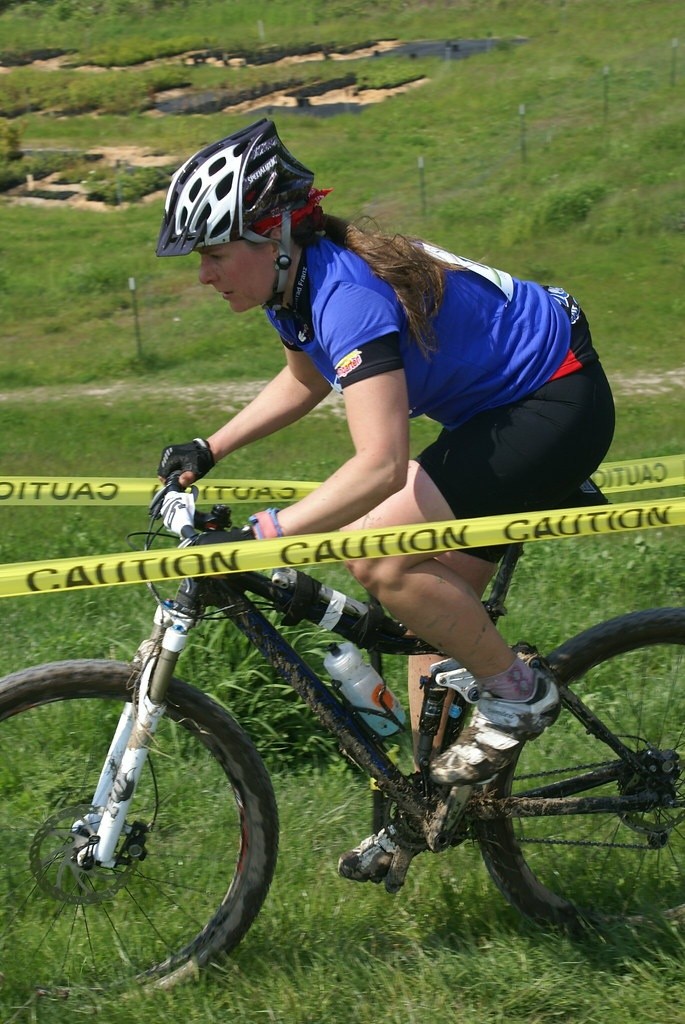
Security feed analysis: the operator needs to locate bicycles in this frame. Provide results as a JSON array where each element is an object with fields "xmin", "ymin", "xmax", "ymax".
[{"xmin": 0, "ymin": 460, "xmax": 685, "ymax": 1006}]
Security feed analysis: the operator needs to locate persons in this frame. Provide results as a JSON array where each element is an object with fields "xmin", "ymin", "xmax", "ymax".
[{"xmin": 155, "ymin": 117, "xmax": 615, "ymax": 882}]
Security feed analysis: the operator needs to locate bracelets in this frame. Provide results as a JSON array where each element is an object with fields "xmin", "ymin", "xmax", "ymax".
[{"xmin": 249, "ymin": 508, "xmax": 284, "ymax": 540}]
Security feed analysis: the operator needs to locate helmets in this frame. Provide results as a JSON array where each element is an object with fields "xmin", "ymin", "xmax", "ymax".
[{"xmin": 154, "ymin": 116, "xmax": 315, "ymax": 258}]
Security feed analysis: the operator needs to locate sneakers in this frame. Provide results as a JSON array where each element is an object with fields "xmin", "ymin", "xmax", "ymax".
[
  {"xmin": 428, "ymin": 668, "xmax": 562, "ymax": 787},
  {"xmin": 337, "ymin": 823, "xmax": 425, "ymax": 883}
]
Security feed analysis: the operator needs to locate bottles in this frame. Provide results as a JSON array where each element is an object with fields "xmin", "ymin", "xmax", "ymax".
[{"xmin": 325, "ymin": 642, "xmax": 405, "ymax": 737}]
